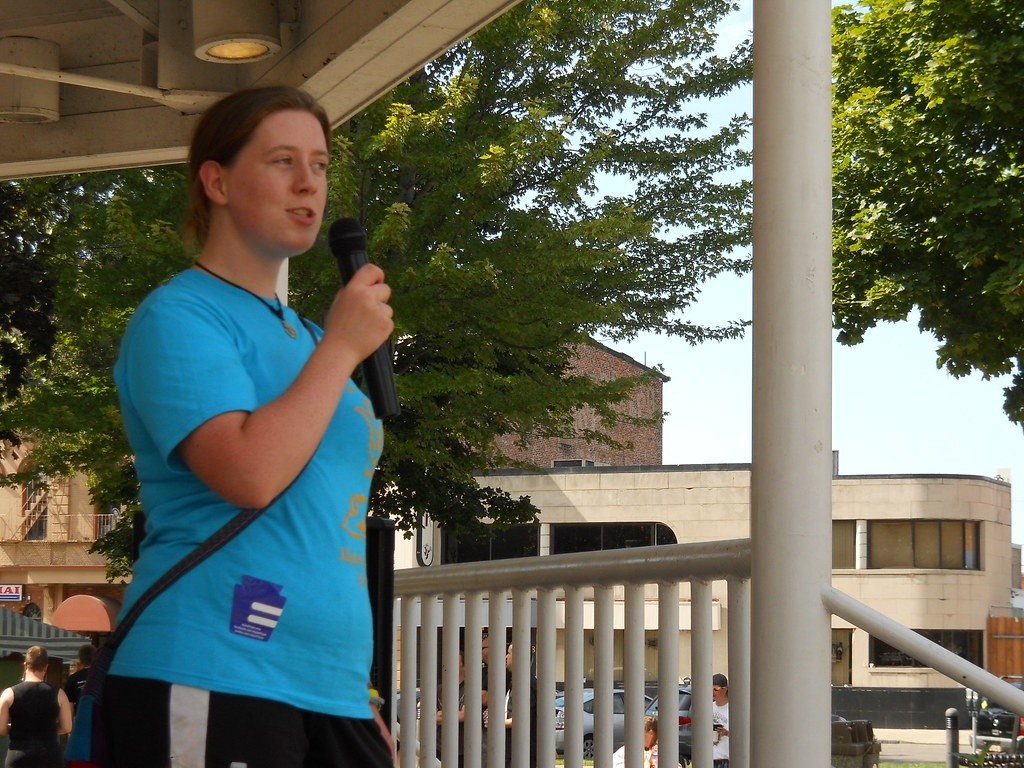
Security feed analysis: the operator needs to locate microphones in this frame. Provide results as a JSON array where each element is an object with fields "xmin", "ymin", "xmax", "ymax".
[{"xmin": 328, "ymin": 217, "xmax": 400, "ymax": 420}]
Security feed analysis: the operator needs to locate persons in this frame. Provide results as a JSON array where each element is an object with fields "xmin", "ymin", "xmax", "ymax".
[
  {"xmin": 713, "ymin": 673, "xmax": 729, "ymax": 768},
  {"xmin": 92, "ymin": 86, "xmax": 395, "ymax": 768},
  {"xmin": 436, "ymin": 630, "xmax": 538, "ymax": 768},
  {"xmin": 643, "ymin": 716, "xmax": 683, "ymax": 768},
  {"xmin": 0, "ymin": 644, "xmax": 97, "ymax": 768}
]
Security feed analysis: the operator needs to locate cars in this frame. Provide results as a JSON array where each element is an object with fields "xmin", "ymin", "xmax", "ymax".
[{"xmin": 555, "ymin": 689, "xmax": 653, "ymax": 760}]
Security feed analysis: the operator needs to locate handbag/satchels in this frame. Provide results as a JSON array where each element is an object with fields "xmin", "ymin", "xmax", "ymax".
[{"xmin": 62, "ymin": 695, "xmax": 112, "ymax": 768}]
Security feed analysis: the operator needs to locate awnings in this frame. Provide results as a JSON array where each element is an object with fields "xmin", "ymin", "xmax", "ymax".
[{"xmin": 50, "ymin": 594, "xmax": 120, "ymax": 633}]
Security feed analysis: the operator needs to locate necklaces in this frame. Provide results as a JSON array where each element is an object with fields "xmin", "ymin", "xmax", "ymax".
[{"xmin": 194, "ymin": 260, "xmax": 297, "ymax": 338}]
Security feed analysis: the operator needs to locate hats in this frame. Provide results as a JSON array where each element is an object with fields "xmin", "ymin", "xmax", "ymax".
[{"xmin": 713, "ymin": 674, "xmax": 727, "ymax": 688}]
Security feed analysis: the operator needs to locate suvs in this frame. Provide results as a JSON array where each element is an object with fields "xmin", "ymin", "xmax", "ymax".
[
  {"xmin": 644, "ymin": 677, "xmax": 692, "ymax": 766},
  {"xmin": 969, "ymin": 680, "xmax": 1024, "ymax": 753}
]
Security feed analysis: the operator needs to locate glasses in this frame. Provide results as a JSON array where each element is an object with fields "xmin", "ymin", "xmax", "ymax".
[{"xmin": 713, "ymin": 687, "xmax": 725, "ymax": 691}]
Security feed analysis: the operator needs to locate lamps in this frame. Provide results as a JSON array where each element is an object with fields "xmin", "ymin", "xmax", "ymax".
[
  {"xmin": 0, "ymin": 35, "xmax": 60, "ymax": 125},
  {"xmin": 192, "ymin": 0, "xmax": 281, "ymax": 63}
]
